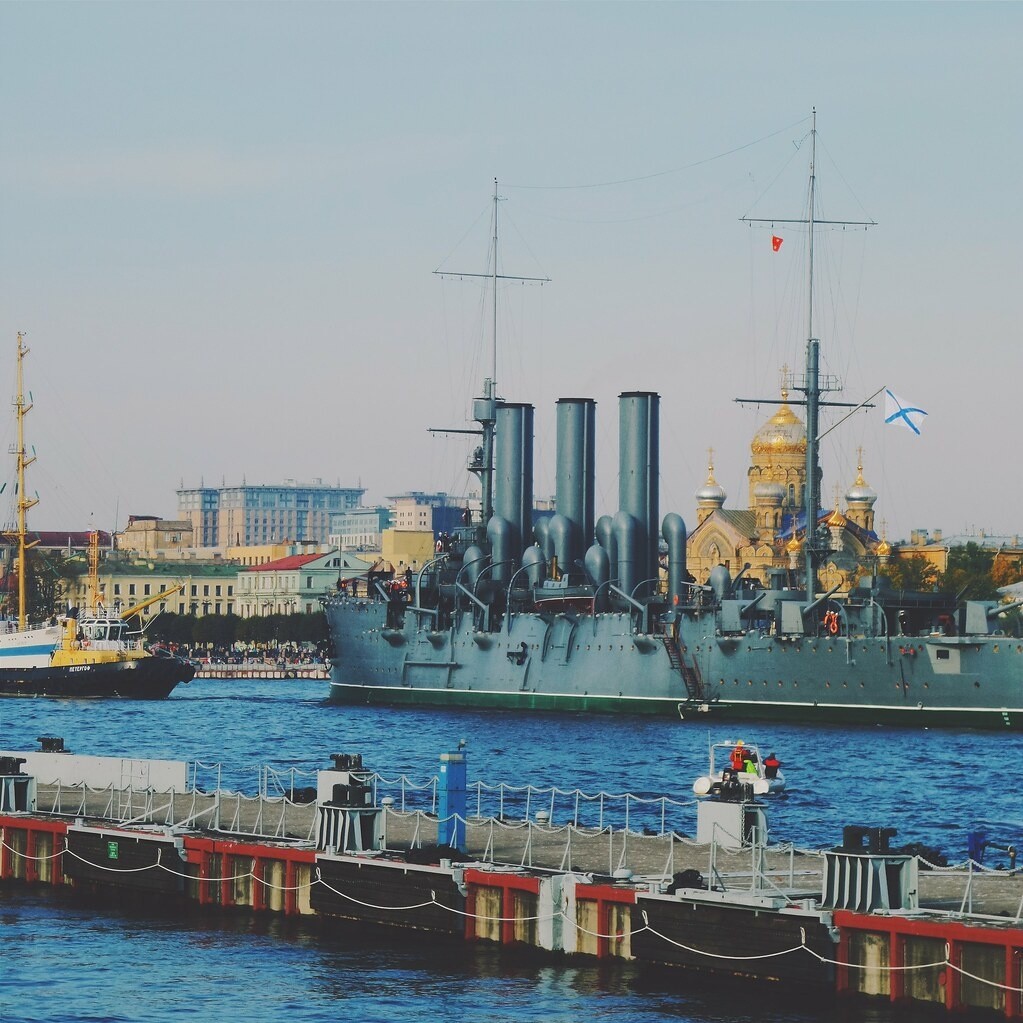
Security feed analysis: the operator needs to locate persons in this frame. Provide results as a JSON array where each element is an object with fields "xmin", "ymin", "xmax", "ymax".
[
  {"xmin": 462, "ymin": 505, "xmax": 471, "ymax": 527},
  {"xmin": 730, "ymin": 739, "xmax": 752, "ymax": 773},
  {"xmin": 337, "ymin": 576, "xmax": 359, "ymax": 597},
  {"xmin": 96, "ymin": 629, "xmax": 103, "ymax": 641},
  {"xmin": 898, "ymin": 612, "xmax": 908, "ymax": 637},
  {"xmin": 762, "ymin": 753, "xmax": 780, "ymax": 780},
  {"xmin": 406, "ymin": 566, "xmax": 412, "ymax": 587},
  {"xmin": 77, "ymin": 631, "xmax": 83, "ymax": 641},
  {"xmin": 386, "ymin": 581, "xmax": 402, "ymax": 601},
  {"xmin": 435, "ymin": 530, "xmax": 450, "ymax": 552},
  {"xmin": 824, "ymin": 610, "xmax": 840, "ymax": 639},
  {"xmin": 147, "ymin": 639, "xmax": 330, "ymax": 666}
]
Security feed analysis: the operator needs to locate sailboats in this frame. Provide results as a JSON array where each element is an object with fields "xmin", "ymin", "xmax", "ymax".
[
  {"xmin": 315, "ymin": 106, "xmax": 1023, "ymax": 732},
  {"xmin": 0, "ymin": 331, "xmax": 195, "ymax": 699}
]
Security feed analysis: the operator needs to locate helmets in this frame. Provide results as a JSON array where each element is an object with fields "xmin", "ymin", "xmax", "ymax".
[{"xmin": 737, "ymin": 740, "xmax": 745, "ymax": 745}]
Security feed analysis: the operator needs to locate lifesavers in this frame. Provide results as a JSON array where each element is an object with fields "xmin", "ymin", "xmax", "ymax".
[
  {"xmin": 830, "ymin": 622, "xmax": 838, "ymax": 633},
  {"xmin": 673, "ymin": 595, "xmax": 679, "ymax": 605}
]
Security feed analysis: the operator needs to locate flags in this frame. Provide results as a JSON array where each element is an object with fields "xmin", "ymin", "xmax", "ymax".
[
  {"xmin": 884, "ymin": 387, "xmax": 929, "ymax": 435},
  {"xmin": 772, "ymin": 235, "xmax": 784, "ymax": 252}
]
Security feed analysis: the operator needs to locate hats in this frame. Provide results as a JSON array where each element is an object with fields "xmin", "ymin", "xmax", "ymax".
[
  {"xmin": 770, "ymin": 753, "xmax": 775, "ymax": 758},
  {"xmin": 826, "ymin": 610, "xmax": 830, "ymax": 613},
  {"xmin": 831, "ymin": 611, "xmax": 834, "ymax": 613}
]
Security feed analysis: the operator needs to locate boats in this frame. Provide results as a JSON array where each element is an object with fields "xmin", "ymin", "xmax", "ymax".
[{"xmin": 692, "ymin": 739, "xmax": 786, "ymax": 795}]
